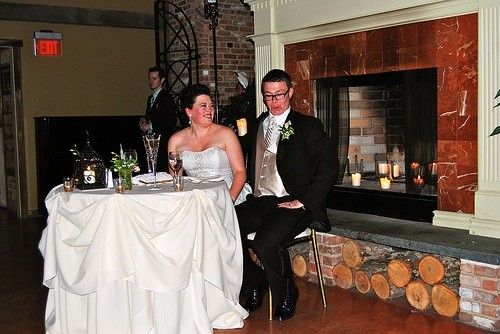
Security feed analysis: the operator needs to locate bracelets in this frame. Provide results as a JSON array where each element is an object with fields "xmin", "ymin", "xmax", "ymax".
[{"xmin": 231, "ymin": 199, "xmax": 235, "ymax": 204}]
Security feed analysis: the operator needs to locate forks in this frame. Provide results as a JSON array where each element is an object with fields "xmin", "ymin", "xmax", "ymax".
[{"xmin": 139, "ymin": 179, "xmax": 172, "ymax": 184}]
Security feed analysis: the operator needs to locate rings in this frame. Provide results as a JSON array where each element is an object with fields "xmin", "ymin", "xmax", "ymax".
[{"xmin": 289, "ymin": 204, "xmax": 290, "ymax": 206}]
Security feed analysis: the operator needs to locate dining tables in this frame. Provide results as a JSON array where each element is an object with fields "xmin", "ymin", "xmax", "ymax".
[{"xmin": 38, "ymin": 176, "xmax": 249, "ymax": 334}]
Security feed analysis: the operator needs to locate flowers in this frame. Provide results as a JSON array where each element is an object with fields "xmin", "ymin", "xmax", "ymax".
[
  {"xmin": 110, "ymin": 144, "xmax": 141, "ymax": 189},
  {"xmin": 278, "ymin": 121, "xmax": 294, "ymax": 140}
]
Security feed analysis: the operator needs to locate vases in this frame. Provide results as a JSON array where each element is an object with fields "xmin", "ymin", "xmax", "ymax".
[{"xmin": 118, "ymin": 170, "xmax": 132, "ymax": 190}]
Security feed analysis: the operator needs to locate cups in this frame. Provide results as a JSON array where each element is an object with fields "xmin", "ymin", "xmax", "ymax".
[
  {"xmin": 173, "ymin": 175, "xmax": 184, "ymax": 191},
  {"xmin": 62, "ymin": 177, "xmax": 75, "ymax": 192},
  {"xmin": 114, "ymin": 179, "xmax": 126, "ymax": 193},
  {"xmin": 123, "ymin": 149, "xmax": 138, "ymax": 162}
]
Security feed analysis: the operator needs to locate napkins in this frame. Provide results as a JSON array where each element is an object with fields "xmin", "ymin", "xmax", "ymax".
[{"xmin": 132, "ymin": 171, "xmax": 173, "ymax": 185}]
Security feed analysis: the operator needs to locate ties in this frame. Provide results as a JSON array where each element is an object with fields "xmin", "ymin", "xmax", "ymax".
[
  {"xmin": 264, "ymin": 119, "xmax": 274, "ymax": 148},
  {"xmin": 151, "ymin": 97, "xmax": 154, "ymax": 107}
]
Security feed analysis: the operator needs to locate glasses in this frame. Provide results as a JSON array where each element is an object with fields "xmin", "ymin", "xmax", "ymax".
[{"xmin": 264, "ymin": 88, "xmax": 290, "ymax": 101}]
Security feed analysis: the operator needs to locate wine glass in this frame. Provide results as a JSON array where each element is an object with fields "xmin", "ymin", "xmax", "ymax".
[
  {"xmin": 168, "ymin": 151, "xmax": 184, "ymax": 188},
  {"xmin": 141, "ymin": 131, "xmax": 162, "ymax": 191}
]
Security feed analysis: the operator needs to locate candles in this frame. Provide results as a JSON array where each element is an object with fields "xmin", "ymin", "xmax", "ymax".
[
  {"xmin": 236, "ymin": 118, "xmax": 247, "ymax": 136},
  {"xmin": 352, "ymin": 173, "xmax": 361, "ymax": 186},
  {"xmin": 108, "ymin": 169, "xmax": 114, "ymax": 188},
  {"xmin": 413, "ymin": 175, "xmax": 423, "ymax": 183},
  {"xmin": 380, "ymin": 177, "xmax": 390, "ymax": 189},
  {"xmin": 394, "ymin": 165, "xmax": 400, "ymax": 177},
  {"xmin": 379, "ymin": 164, "xmax": 387, "ymax": 174},
  {"xmin": 84, "ymin": 166, "xmax": 95, "ymax": 183}
]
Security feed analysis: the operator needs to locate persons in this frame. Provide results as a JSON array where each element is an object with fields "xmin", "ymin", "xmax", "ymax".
[
  {"xmin": 139, "ymin": 67, "xmax": 177, "ymax": 171},
  {"xmin": 167, "ymin": 82, "xmax": 253, "ymax": 208},
  {"xmin": 233, "ymin": 68, "xmax": 341, "ymax": 321}
]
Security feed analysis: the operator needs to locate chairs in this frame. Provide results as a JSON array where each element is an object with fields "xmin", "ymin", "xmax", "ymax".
[{"xmin": 244, "ymin": 228, "xmax": 327, "ymax": 321}]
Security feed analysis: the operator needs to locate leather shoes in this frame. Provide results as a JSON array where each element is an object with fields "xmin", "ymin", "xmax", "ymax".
[
  {"xmin": 276, "ymin": 290, "xmax": 300, "ymax": 321},
  {"xmin": 245, "ymin": 286, "xmax": 267, "ymax": 312}
]
